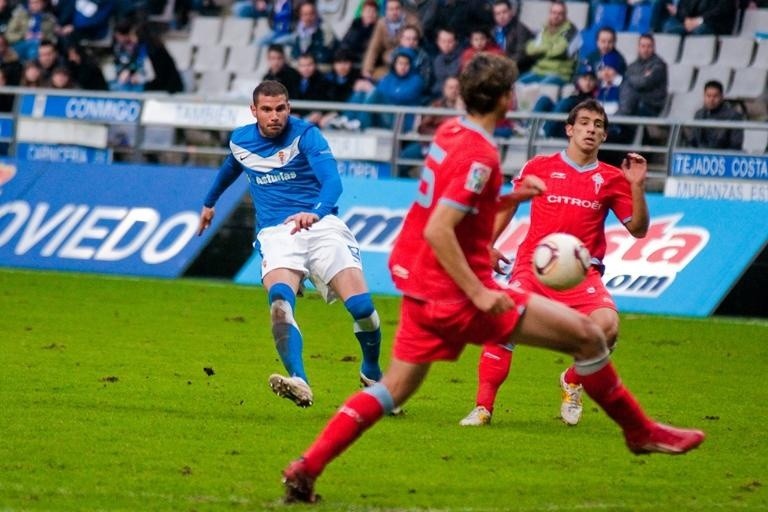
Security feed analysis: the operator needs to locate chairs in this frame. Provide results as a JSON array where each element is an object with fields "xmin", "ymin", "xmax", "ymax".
[
  {"xmin": 162, "ymin": 1, "xmax": 358, "ymax": 99},
  {"xmin": 517, "ymin": 2, "xmax": 767, "ymax": 155}
]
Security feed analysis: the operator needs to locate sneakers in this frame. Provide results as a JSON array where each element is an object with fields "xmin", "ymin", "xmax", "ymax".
[
  {"xmin": 280, "ymin": 457, "xmax": 322, "ymax": 506},
  {"xmin": 458, "ymin": 404, "xmax": 493, "ymax": 428},
  {"xmin": 268, "ymin": 373, "xmax": 314, "ymax": 409},
  {"xmin": 558, "ymin": 366, "xmax": 584, "ymax": 427},
  {"xmin": 357, "ymin": 369, "xmax": 406, "ymax": 418},
  {"xmin": 622, "ymin": 416, "xmax": 706, "ymax": 456}
]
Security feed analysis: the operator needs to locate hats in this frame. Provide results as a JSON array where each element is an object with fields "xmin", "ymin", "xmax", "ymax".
[
  {"xmin": 577, "ymin": 65, "xmax": 598, "ymax": 78},
  {"xmin": 596, "ymin": 54, "xmax": 622, "ymax": 75}
]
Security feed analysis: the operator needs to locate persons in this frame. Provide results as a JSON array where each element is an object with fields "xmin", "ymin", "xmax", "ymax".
[
  {"xmin": 280, "ymin": 50, "xmax": 707, "ymax": 504},
  {"xmin": 198, "ymin": 79, "xmax": 403, "ymax": 418},
  {"xmin": 458, "ymin": 99, "xmax": 649, "ymax": 427}
]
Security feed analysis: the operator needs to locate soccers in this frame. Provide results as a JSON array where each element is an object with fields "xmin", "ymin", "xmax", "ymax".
[{"xmin": 531, "ymin": 234, "xmax": 592, "ymax": 290}]
